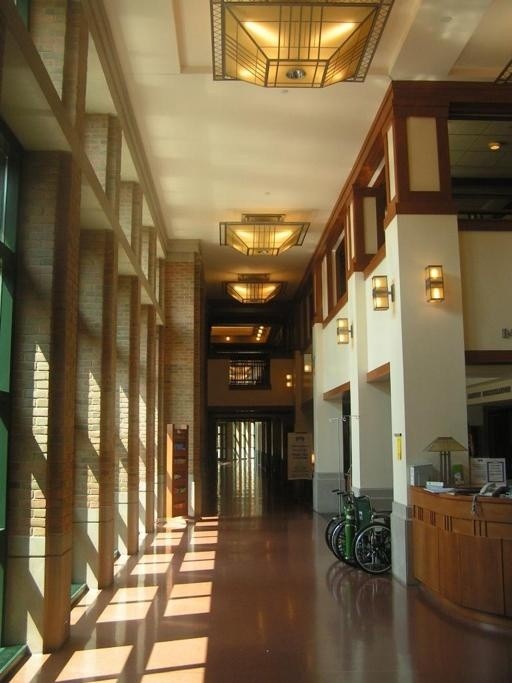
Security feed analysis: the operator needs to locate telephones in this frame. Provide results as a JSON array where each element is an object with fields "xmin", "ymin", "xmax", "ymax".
[{"xmin": 479, "ymin": 482, "xmax": 506, "ymax": 497}]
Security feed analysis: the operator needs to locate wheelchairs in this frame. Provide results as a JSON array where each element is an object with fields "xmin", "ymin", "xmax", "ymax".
[{"xmin": 321, "ymin": 485, "xmax": 392, "ymax": 573}]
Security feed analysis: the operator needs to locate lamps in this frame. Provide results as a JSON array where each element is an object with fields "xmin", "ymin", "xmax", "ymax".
[
  {"xmin": 219, "ymin": 212, "xmax": 311, "ymax": 304},
  {"xmin": 211, "ymin": 0, "xmax": 396, "ymax": 88},
  {"xmin": 421, "ymin": 436, "xmax": 467, "ymax": 485},
  {"xmin": 337, "ymin": 264, "xmax": 445, "ymax": 344},
  {"xmin": 303, "ymin": 352, "xmax": 315, "ymax": 372}
]
